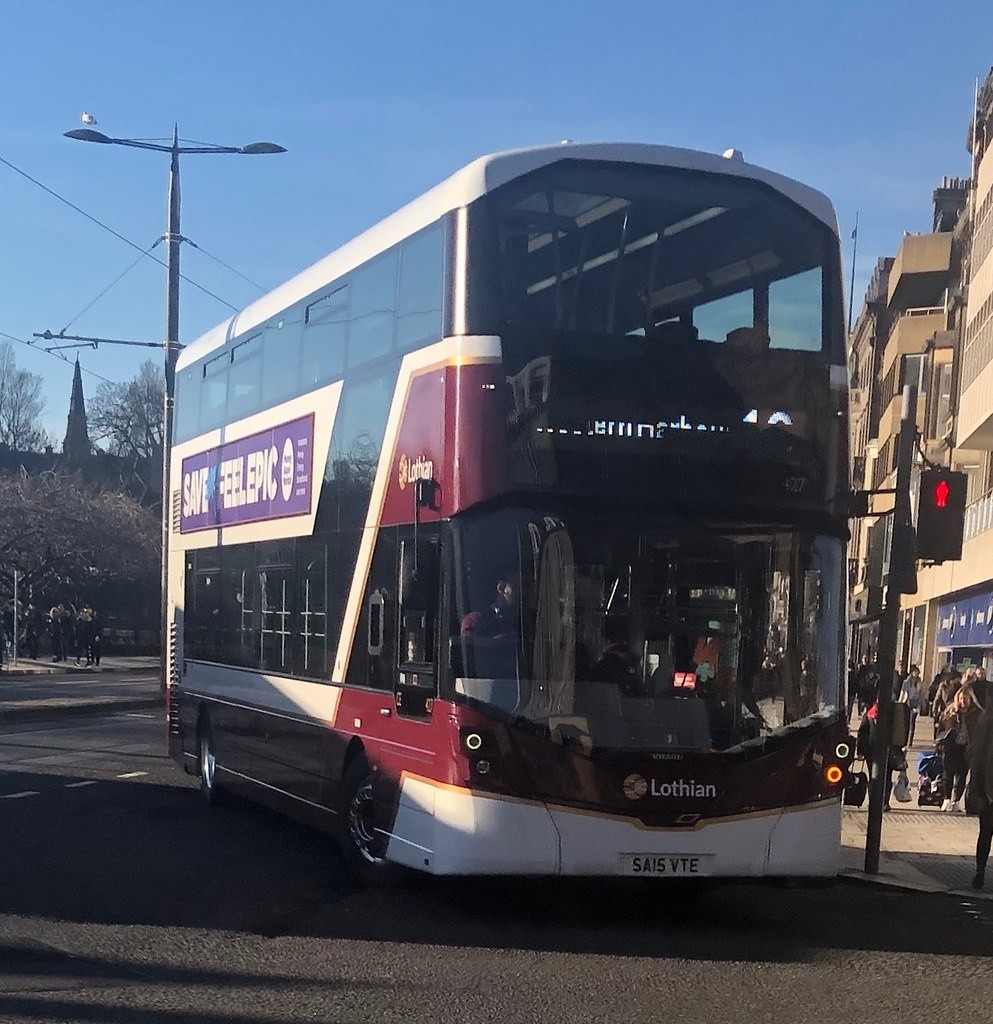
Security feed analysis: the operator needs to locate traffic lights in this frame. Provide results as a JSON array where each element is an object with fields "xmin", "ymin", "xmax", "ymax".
[{"xmin": 918, "ymin": 469, "xmax": 967, "ymax": 561}]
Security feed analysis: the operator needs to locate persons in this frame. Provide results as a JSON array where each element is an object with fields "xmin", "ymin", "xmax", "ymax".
[
  {"xmin": 0, "ymin": 599, "xmax": 103, "ymax": 668},
  {"xmin": 845, "ymin": 652, "xmax": 992, "ymax": 892},
  {"xmin": 764, "ymin": 622, "xmax": 819, "ymax": 716},
  {"xmin": 461, "ymin": 520, "xmax": 627, "ymax": 686}
]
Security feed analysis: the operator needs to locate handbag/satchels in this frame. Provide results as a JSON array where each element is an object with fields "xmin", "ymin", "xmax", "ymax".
[{"xmin": 934, "ymin": 728, "xmax": 958, "ymax": 752}]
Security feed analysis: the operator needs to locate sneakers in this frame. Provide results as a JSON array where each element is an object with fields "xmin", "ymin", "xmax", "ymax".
[{"xmin": 941, "ymin": 798, "xmax": 963, "ymax": 812}]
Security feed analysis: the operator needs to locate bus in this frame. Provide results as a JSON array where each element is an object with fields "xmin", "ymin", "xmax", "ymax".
[{"xmin": 167, "ymin": 139, "xmax": 851, "ymax": 877}]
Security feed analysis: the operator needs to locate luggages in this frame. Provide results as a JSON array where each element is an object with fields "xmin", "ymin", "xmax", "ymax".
[
  {"xmin": 843, "ymin": 770, "xmax": 868, "ymax": 806},
  {"xmin": 919, "ymin": 754, "xmax": 945, "ymax": 808}
]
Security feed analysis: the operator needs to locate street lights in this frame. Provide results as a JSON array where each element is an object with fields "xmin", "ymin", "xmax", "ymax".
[{"xmin": 64, "ymin": 129, "xmax": 289, "ymax": 694}]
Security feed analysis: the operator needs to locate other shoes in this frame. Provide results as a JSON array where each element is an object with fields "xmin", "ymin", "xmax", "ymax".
[{"xmin": 973, "ymin": 871, "xmax": 983, "ymax": 889}]
